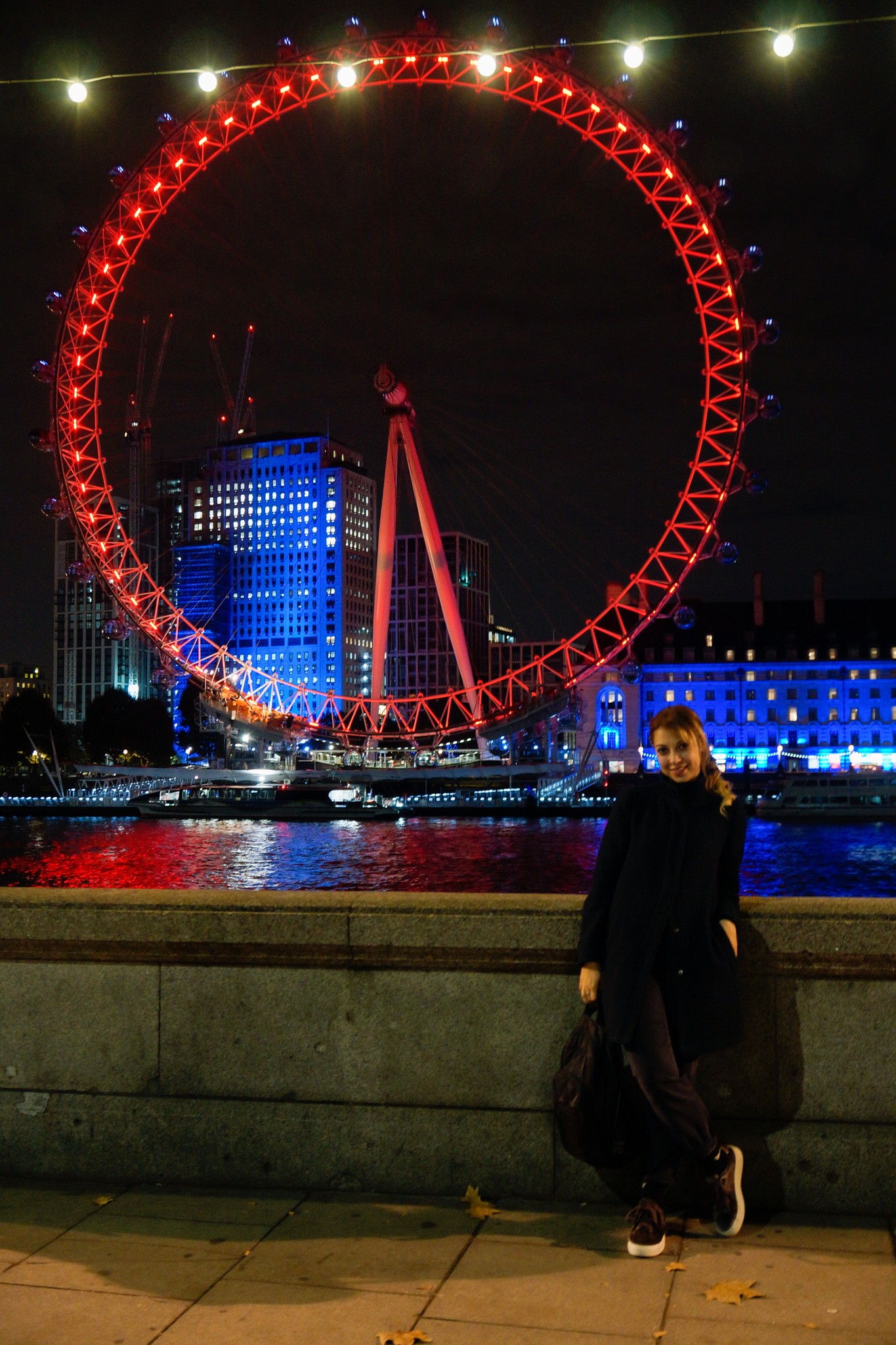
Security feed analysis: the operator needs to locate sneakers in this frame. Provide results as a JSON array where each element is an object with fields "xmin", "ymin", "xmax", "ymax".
[
  {"xmin": 626, "ymin": 1198, "xmax": 666, "ymax": 1258},
  {"xmin": 707, "ymin": 1143, "xmax": 746, "ymax": 1237}
]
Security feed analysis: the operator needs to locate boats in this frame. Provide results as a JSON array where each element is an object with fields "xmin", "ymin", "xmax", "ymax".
[{"xmin": 751, "ymin": 769, "xmax": 895, "ymax": 819}]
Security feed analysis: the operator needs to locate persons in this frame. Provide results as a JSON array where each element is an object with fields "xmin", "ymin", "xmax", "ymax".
[{"xmin": 576, "ymin": 705, "xmax": 747, "ymax": 1258}]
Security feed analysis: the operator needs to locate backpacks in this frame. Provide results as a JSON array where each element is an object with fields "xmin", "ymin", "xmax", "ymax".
[{"xmin": 550, "ymin": 1006, "xmax": 641, "ymax": 1170}]
